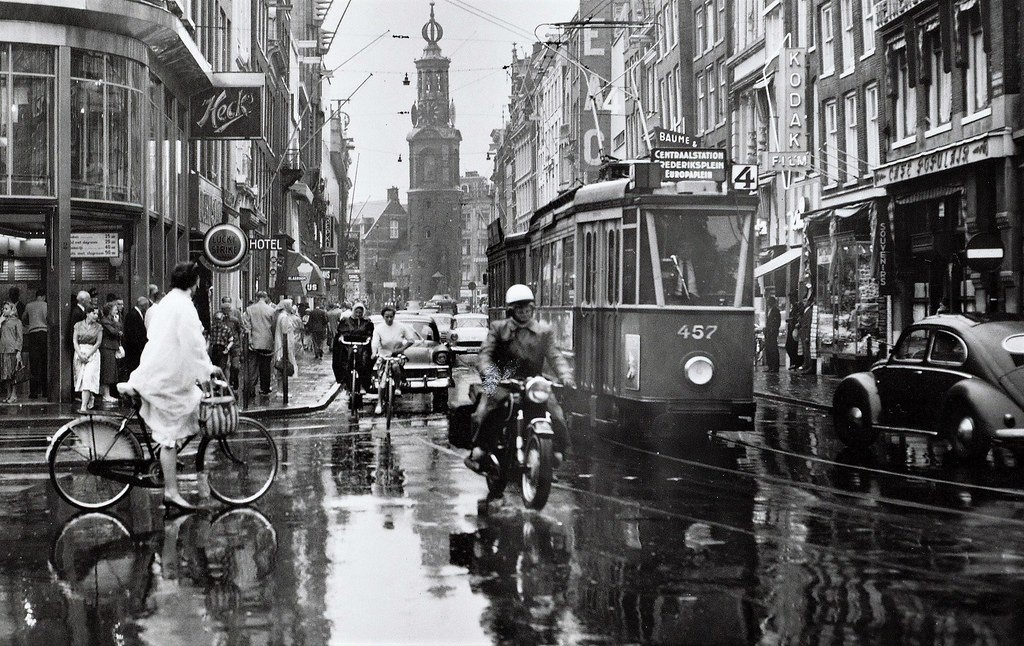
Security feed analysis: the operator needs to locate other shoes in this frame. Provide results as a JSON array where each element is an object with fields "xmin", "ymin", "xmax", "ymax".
[
  {"xmin": 87, "ymin": 395, "xmax": 94, "ymax": 408},
  {"xmin": 470, "ymin": 446, "xmax": 486, "ymax": 471},
  {"xmin": 80, "ymin": 404, "xmax": 87, "ymax": 412},
  {"xmin": 360, "ymin": 390, "xmax": 367, "ymax": 394},
  {"xmin": 394, "ymin": 387, "xmax": 401, "ymax": 395},
  {"xmin": 375, "ymin": 405, "xmax": 382, "ymax": 414},
  {"xmin": 763, "ymin": 363, "xmax": 816, "ymax": 375}
]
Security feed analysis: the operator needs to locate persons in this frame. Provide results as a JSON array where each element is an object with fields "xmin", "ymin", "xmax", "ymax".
[
  {"xmin": 332, "ymin": 304, "xmax": 374, "ymax": 395},
  {"xmin": 798, "ymin": 298, "xmax": 814, "ymax": 371},
  {"xmin": 470, "ymin": 284, "xmax": 577, "ymax": 462},
  {"xmin": 0, "ymin": 286, "xmax": 50, "ymax": 404},
  {"xmin": 370, "ymin": 307, "xmax": 416, "ymax": 414},
  {"xmin": 764, "ymin": 297, "xmax": 781, "ymax": 373},
  {"xmin": 68, "ymin": 290, "xmax": 167, "ymax": 413},
  {"xmin": 240, "ymin": 292, "xmax": 304, "ymax": 396},
  {"xmin": 786, "ymin": 290, "xmax": 803, "ymax": 371},
  {"xmin": 207, "ymin": 296, "xmax": 243, "ymax": 393},
  {"xmin": 304, "ymin": 301, "xmax": 329, "ymax": 360},
  {"xmin": 664, "ymin": 231, "xmax": 709, "ymax": 305},
  {"xmin": 130, "ymin": 262, "xmax": 223, "ymax": 514},
  {"xmin": 297, "ymin": 301, "xmax": 353, "ymax": 351}
]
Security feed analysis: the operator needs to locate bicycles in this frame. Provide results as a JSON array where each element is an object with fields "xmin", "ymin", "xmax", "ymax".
[
  {"xmin": 340, "ymin": 336, "xmax": 372, "ymax": 416},
  {"xmin": 372, "ymin": 354, "xmax": 408, "ymax": 431},
  {"xmin": 46, "ymin": 371, "xmax": 286, "ymax": 510}
]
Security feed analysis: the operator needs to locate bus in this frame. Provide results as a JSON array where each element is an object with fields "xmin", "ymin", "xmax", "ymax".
[{"xmin": 482, "ymin": 67, "xmax": 762, "ymax": 439}]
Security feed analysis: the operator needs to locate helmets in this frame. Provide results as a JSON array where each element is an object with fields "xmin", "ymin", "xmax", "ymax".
[{"xmin": 506, "ymin": 283, "xmax": 534, "ymax": 304}]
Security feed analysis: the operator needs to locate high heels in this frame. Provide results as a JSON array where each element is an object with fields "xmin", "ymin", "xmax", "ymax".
[
  {"xmin": 162, "ymin": 495, "xmax": 209, "ymax": 516},
  {"xmin": 6, "ymin": 395, "xmax": 18, "ymax": 403},
  {"xmin": 156, "ymin": 448, "xmax": 186, "ymax": 467},
  {"xmin": 3, "ymin": 396, "xmax": 10, "ymax": 402}
]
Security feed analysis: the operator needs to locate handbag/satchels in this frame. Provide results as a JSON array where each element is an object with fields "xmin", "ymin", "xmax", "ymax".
[
  {"xmin": 11, "ymin": 360, "xmax": 32, "ymax": 385},
  {"xmin": 200, "ymin": 371, "xmax": 237, "ymax": 437},
  {"xmin": 276, "ymin": 357, "xmax": 296, "ymax": 376},
  {"xmin": 115, "ymin": 336, "xmax": 126, "ymax": 360}
]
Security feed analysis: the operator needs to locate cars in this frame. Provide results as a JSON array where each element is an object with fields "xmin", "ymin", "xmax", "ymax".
[
  {"xmin": 831, "ymin": 317, "xmax": 1024, "ymax": 467},
  {"xmin": 370, "ymin": 294, "xmax": 489, "ymax": 405}
]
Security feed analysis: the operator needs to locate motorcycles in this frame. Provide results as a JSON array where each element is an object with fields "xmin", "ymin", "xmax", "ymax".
[{"xmin": 467, "ymin": 378, "xmax": 572, "ymax": 511}]
